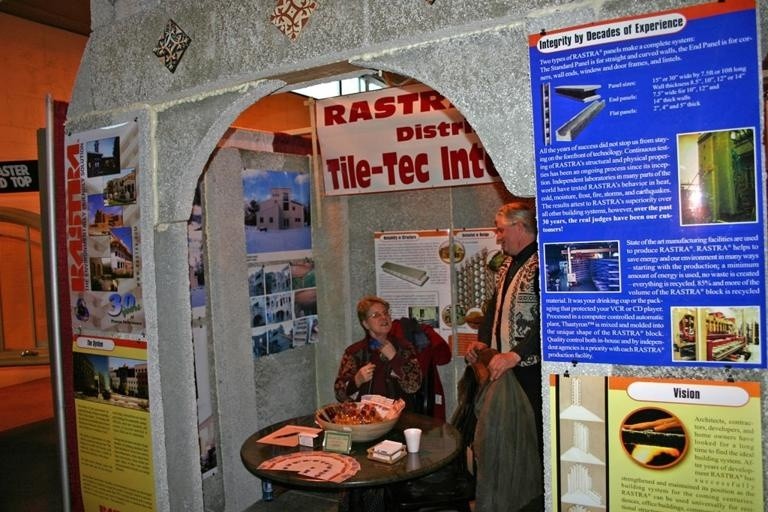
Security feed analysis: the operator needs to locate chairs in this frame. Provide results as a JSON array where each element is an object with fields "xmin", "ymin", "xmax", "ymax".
[{"xmin": 392, "ymin": 319, "xmax": 433, "ymax": 416}]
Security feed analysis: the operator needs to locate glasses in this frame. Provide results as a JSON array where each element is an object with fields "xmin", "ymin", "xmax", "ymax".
[{"xmin": 367, "ymin": 308, "xmax": 392, "ymax": 320}]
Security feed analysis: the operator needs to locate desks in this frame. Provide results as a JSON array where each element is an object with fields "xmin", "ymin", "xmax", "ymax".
[{"xmin": 238, "ymin": 414, "xmax": 466, "ymax": 511}]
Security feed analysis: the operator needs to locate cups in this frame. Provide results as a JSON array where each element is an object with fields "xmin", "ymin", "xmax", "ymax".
[{"xmin": 404, "ymin": 427, "xmax": 422, "ymax": 453}]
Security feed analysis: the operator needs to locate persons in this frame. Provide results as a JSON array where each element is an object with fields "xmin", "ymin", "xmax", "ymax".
[
  {"xmin": 461, "ymin": 201, "xmax": 544, "ymax": 511},
  {"xmin": 333, "ymin": 294, "xmax": 424, "ymax": 419}
]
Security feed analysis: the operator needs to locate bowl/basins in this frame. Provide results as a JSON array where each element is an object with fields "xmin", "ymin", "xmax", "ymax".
[{"xmin": 315, "ymin": 401, "xmax": 402, "ymax": 442}]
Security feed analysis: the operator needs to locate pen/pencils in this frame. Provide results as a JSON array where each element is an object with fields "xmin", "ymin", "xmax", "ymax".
[{"xmin": 275, "ymin": 432, "xmax": 300, "ymax": 438}]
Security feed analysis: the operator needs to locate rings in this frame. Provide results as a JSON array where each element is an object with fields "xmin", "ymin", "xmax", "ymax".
[{"xmin": 366, "ymin": 370, "xmax": 370, "ymax": 374}]
